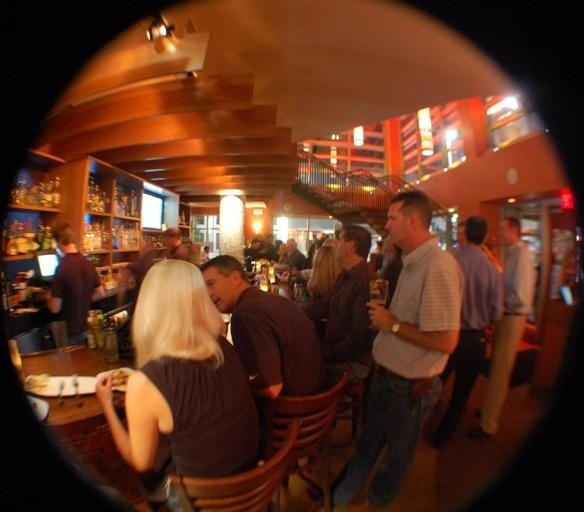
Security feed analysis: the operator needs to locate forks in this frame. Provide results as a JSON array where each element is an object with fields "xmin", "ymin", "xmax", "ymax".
[{"xmin": 71, "ymin": 378, "xmax": 80, "ymax": 400}]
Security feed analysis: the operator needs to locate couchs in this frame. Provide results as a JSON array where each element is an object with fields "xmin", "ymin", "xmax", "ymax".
[{"xmin": 485, "ymin": 321, "xmax": 538, "ymax": 386}]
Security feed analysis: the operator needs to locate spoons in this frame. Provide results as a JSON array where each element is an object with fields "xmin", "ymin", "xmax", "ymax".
[{"xmin": 55, "ymin": 381, "xmax": 65, "ymax": 406}]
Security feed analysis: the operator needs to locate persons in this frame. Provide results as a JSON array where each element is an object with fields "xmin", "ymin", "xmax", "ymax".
[
  {"xmin": 325, "ymin": 191, "xmax": 462, "ymax": 509},
  {"xmin": 307, "ymin": 245, "xmax": 341, "ymax": 300},
  {"xmin": 36, "ymin": 221, "xmax": 106, "ymax": 348},
  {"xmin": 95, "ymin": 257, "xmax": 257, "ymax": 512},
  {"xmin": 369, "ymin": 235, "xmax": 402, "ymax": 283},
  {"xmin": 199, "ymin": 254, "xmax": 326, "ymax": 443},
  {"xmin": 241, "ymin": 238, "xmax": 307, "ymax": 280},
  {"xmin": 431, "ymin": 215, "xmax": 506, "ymax": 448},
  {"xmin": 136, "ymin": 225, "xmax": 210, "ymax": 264},
  {"xmin": 297, "ymin": 225, "xmax": 383, "ymax": 364},
  {"xmin": 462, "ymin": 217, "xmax": 537, "ymax": 441},
  {"xmin": 307, "ymin": 230, "xmax": 340, "ymax": 247},
  {"xmin": 307, "ymin": 247, "xmax": 315, "ymax": 270}
]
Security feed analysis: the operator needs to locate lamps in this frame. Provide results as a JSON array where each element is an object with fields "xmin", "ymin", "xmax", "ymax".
[
  {"xmin": 185, "ymin": 70, "xmax": 202, "ymax": 79},
  {"xmin": 417, "ymin": 107, "xmax": 434, "ymax": 156},
  {"xmin": 329, "ymin": 144, "xmax": 337, "ymax": 179},
  {"xmin": 146, "ymin": 13, "xmax": 176, "ymax": 55},
  {"xmin": 354, "ymin": 126, "xmax": 364, "ymax": 146}
]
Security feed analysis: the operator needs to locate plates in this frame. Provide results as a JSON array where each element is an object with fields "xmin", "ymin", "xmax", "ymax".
[
  {"xmin": 24, "ymin": 376, "xmax": 97, "ymax": 397},
  {"xmin": 94, "ymin": 365, "xmax": 134, "ymax": 393},
  {"xmin": 25, "ymin": 394, "xmax": 50, "ymax": 422}
]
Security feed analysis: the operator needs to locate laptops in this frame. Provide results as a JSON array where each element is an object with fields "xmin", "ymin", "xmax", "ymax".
[{"xmin": 36, "ymin": 250, "xmax": 65, "ymax": 283}]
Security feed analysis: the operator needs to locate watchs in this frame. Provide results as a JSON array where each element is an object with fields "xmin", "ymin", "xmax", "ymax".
[{"xmin": 388, "ymin": 319, "xmax": 404, "ymax": 336}]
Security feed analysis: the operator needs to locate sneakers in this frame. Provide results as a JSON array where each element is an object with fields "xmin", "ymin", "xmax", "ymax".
[
  {"xmin": 308, "ymin": 486, "xmax": 333, "ymax": 502},
  {"xmin": 468, "ymin": 426, "xmax": 493, "ymax": 439}
]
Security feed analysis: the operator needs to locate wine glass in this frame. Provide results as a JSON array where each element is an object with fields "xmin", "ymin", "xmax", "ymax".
[{"xmin": 368, "ymin": 280, "xmax": 388, "ymax": 332}]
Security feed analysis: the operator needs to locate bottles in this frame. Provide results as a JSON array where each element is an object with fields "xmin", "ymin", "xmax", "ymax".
[
  {"xmin": 257, "ymin": 263, "xmax": 271, "ymax": 294},
  {"xmin": 115, "ymin": 189, "xmax": 137, "ymax": 217},
  {"xmin": 86, "ymin": 180, "xmax": 110, "ymax": 213},
  {"xmin": 82, "ymin": 221, "xmax": 108, "ymax": 251},
  {"xmin": 104, "ymin": 320, "xmax": 119, "ymax": 362},
  {"xmin": 85, "ymin": 310, "xmax": 96, "ymax": 349},
  {"xmin": 143, "ymin": 232, "xmax": 164, "ymax": 248},
  {"xmin": 8, "ymin": 174, "xmax": 61, "ymax": 208},
  {"xmin": 94, "ymin": 310, "xmax": 105, "ymax": 349},
  {"xmin": 0, "ymin": 269, "xmax": 30, "ymax": 312},
  {"xmin": 2, "ymin": 219, "xmax": 55, "ymax": 258},
  {"xmin": 288, "ymin": 273, "xmax": 306, "ymax": 301},
  {"xmin": 112, "ymin": 222, "xmax": 139, "ymax": 249}
]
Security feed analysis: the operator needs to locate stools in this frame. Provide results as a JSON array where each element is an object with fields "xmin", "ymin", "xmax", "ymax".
[{"xmin": 338, "ymin": 378, "xmax": 366, "ymax": 441}]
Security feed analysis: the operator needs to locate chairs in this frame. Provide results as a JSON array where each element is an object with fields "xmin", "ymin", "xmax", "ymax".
[
  {"xmin": 164, "ymin": 418, "xmax": 303, "ymax": 512},
  {"xmin": 259, "ymin": 372, "xmax": 350, "ymax": 511}
]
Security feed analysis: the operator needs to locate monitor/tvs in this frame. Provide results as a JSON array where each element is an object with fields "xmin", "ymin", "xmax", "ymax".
[{"xmin": 140, "ymin": 189, "xmax": 165, "ymax": 230}]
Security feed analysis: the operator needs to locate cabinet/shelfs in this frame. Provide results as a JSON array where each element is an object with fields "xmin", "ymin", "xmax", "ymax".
[
  {"xmin": 178, "ymin": 201, "xmax": 192, "ymax": 245},
  {"xmin": 142, "ymin": 228, "xmax": 168, "ymax": 263},
  {"xmin": 61, "ymin": 153, "xmax": 143, "ymax": 302},
  {"xmin": 2, "ymin": 147, "xmax": 68, "ymax": 321}
]
